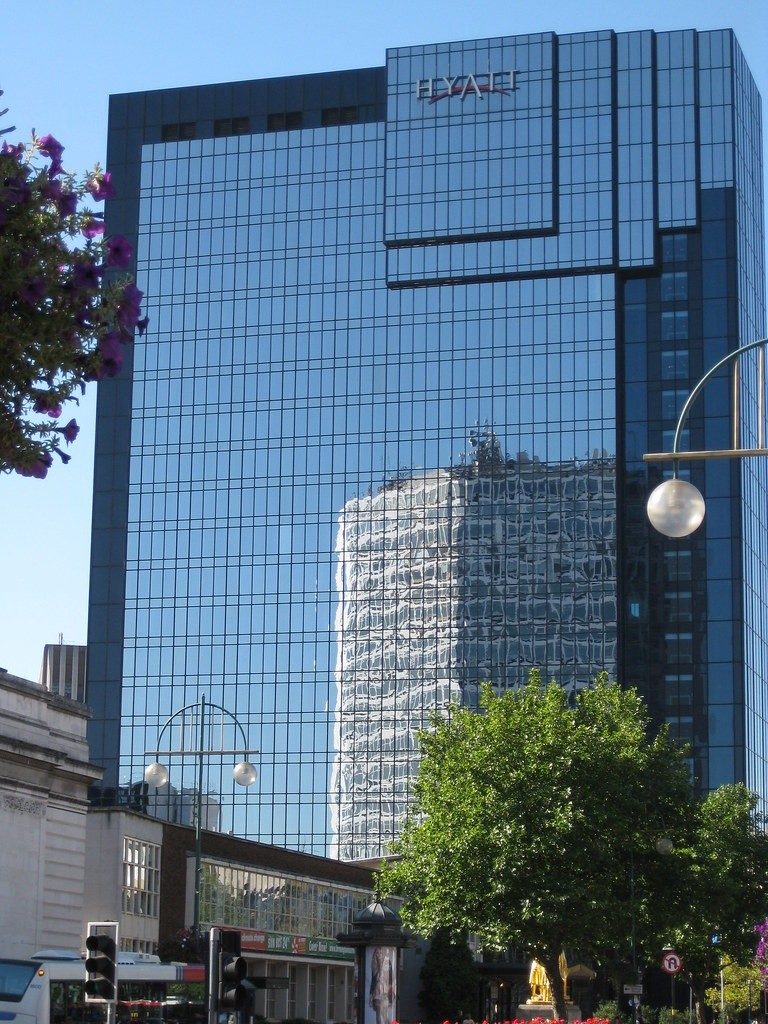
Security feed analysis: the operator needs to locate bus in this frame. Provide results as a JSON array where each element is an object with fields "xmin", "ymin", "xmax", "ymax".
[{"xmin": 2, "ymin": 950, "xmax": 256, "ymax": 1024}]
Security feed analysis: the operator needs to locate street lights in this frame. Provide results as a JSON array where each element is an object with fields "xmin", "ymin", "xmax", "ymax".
[
  {"xmin": 627, "ymin": 796, "xmax": 675, "ymax": 970},
  {"xmin": 644, "ymin": 337, "xmax": 767, "ymax": 539},
  {"xmin": 220, "ymin": 952, "xmax": 250, "ymax": 1011},
  {"xmin": 85, "ymin": 922, "xmax": 119, "ymax": 1003},
  {"xmin": 144, "ymin": 693, "xmax": 259, "ymax": 933}
]
